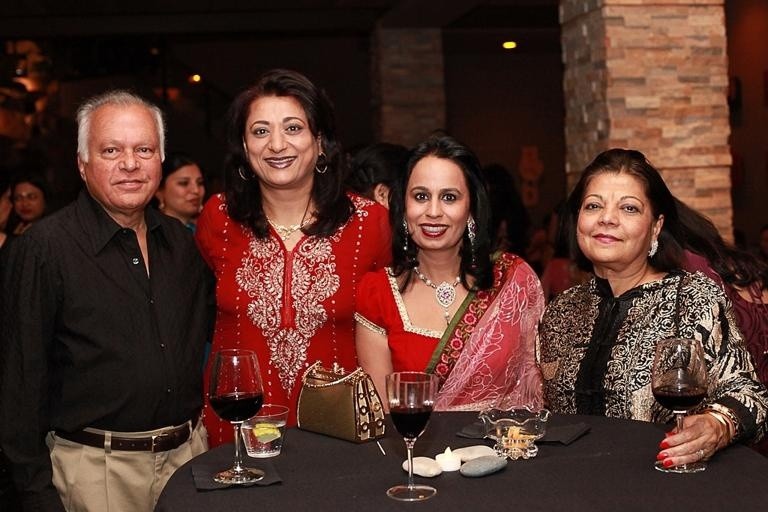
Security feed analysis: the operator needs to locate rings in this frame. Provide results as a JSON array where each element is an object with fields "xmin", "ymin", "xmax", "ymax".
[{"xmin": 697, "ymin": 450, "xmax": 703, "ymax": 461}]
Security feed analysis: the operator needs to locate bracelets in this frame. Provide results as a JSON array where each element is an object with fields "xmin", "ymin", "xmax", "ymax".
[
  {"xmin": 709, "ymin": 411, "xmax": 731, "ymax": 445},
  {"xmin": 695, "ymin": 403, "xmax": 740, "ymax": 441}
]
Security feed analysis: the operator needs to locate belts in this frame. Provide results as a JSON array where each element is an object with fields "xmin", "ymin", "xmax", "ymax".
[{"xmin": 57, "ymin": 416, "xmax": 200, "ymax": 452}]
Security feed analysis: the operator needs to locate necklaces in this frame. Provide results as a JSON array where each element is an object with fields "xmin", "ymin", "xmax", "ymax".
[{"xmin": 413, "ymin": 264, "xmax": 461, "ymax": 326}]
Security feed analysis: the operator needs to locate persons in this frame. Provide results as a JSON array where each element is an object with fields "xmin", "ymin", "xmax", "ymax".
[
  {"xmin": 537, "ymin": 148, "xmax": 766, "ymax": 469},
  {"xmin": 1, "ymin": 72, "xmax": 592, "ymax": 305},
  {"xmin": 191, "ymin": 69, "xmax": 394, "ymax": 453},
  {"xmin": 2, "ymin": 92, "xmax": 219, "ymax": 512},
  {"xmin": 356, "ymin": 130, "xmax": 542, "ymax": 412}
]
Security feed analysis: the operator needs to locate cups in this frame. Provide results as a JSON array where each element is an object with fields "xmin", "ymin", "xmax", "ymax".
[
  {"xmin": 478, "ymin": 406, "xmax": 551, "ymax": 460},
  {"xmin": 240, "ymin": 405, "xmax": 290, "ymax": 459}
]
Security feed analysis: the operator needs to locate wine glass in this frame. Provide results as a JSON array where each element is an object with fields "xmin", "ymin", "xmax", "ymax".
[
  {"xmin": 385, "ymin": 372, "xmax": 437, "ymax": 503},
  {"xmin": 652, "ymin": 339, "xmax": 708, "ymax": 474},
  {"xmin": 208, "ymin": 350, "xmax": 265, "ymax": 486}
]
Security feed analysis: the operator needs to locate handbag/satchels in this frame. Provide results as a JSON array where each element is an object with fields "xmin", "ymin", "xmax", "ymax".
[{"xmin": 297, "ymin": 362, "xmax": 386, "ymax": 443}]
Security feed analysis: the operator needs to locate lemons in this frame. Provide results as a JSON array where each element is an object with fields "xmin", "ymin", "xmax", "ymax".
[{"xmin": 251, "ymin": 422, "xmax": 281, "ymax": 446}]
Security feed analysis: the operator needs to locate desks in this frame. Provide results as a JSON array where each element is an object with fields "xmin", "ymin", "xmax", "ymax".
[{"xmin": 154, "ymin": 411, "xmax": 768, "ymax": 512}]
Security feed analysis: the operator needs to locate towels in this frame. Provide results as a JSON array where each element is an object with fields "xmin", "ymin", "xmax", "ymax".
[
  {"xmin": 456, "ymin": 422, "xmax": 592, "ymax": 445},
  {"xmin": 191, "ymin": 459, "xmax": 283, "ymax": 491}
]
